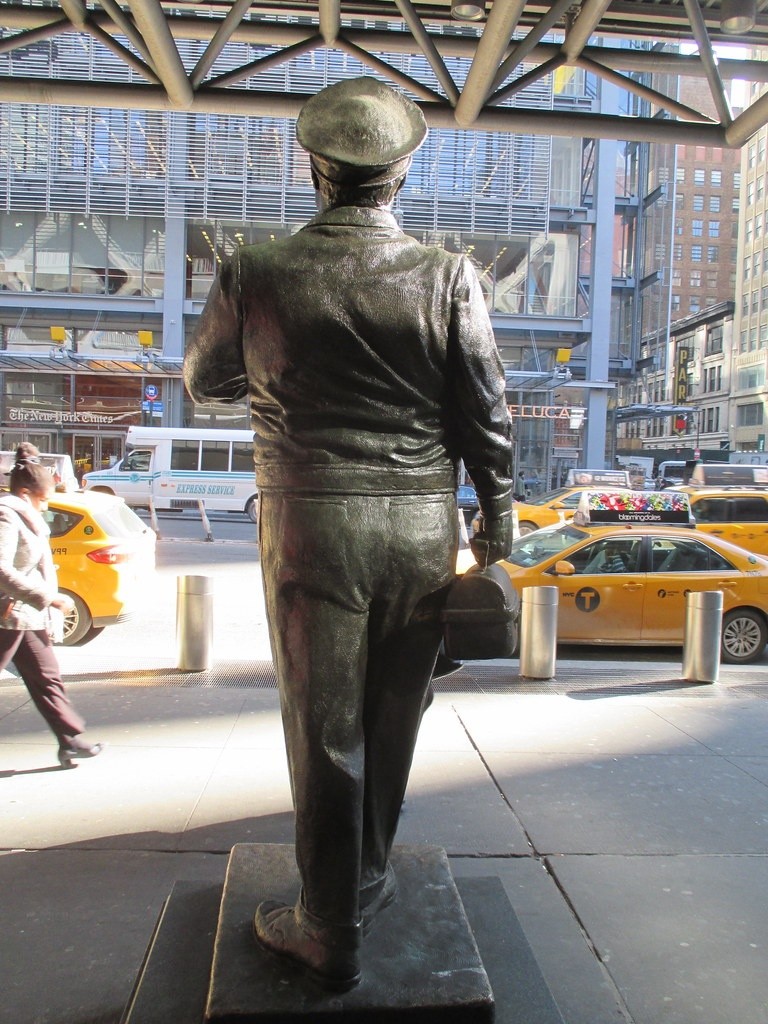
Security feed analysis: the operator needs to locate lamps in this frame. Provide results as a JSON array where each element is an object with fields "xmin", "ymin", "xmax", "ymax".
[
  {"xmin": 719, "ymin": 0, "xmax": 756, "ymax": 34},
  {"xmin": 451, "ymin": 0, "xmax": 486, "ymax": 21},
  {"xmin": 50, "ymin": 326, "xmax": 67, "ymax": 353},
  {"xmin": 137, "ymin": 330, "xmax": 153, "ymax": 354},
  {"xmin": 556, "ymin": 348, "xmax": 572, "ymax": 368}
]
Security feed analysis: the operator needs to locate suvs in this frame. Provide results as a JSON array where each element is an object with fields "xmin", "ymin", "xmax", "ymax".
[{"xmin": 0, "ymin": 488, "xmax": 158, "ymax": 646}]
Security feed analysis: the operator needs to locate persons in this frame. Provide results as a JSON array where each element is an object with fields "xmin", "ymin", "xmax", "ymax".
[
  {"xmin": 592, "ymin": 543, "xmax": 629, "ymax": 573},
  {"xmin": 0, "ymin": 442, "xmax": 105, "ymax": 769},
  {"xmin": 181, "ymin": 76, "xmax": 515, "ymax": 993},
  {"xmin": 516, "ymin": 471, "xmax": 526, "ymax": 502}
]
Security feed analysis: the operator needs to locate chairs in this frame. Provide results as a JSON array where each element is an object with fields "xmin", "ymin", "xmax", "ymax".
[{"xmin": 628, "ymin": 541, "xmax": 641, "ymax": 572}]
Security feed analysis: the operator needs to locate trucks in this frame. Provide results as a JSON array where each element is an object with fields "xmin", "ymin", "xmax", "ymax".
[{"xmin": 616, "ymin": 456, "xmax": 655, "ymax": 486}]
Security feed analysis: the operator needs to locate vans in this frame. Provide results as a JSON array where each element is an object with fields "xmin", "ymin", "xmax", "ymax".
[
  {"xmin": 81, "ymin": 426, "xmax": 260, "ymax": 522},
  {"xmin": 657, "ymin": 460, "xmax": 688, "ymax": 487}
]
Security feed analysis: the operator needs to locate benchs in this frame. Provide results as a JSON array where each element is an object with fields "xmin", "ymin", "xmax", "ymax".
[{"xmin": 657, "ymin": 547, "xmax": 727, "ymax": 572}]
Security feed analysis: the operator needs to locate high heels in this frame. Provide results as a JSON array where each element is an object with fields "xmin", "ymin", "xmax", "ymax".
[{"xmin": 57, "ymin": 742, "xmax": 106, "ymax": 770}]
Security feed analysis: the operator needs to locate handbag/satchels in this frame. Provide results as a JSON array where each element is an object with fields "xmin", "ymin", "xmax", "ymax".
[{"xmin": 0, "ymin": 598, "xmax": 19, "ymax": 620}]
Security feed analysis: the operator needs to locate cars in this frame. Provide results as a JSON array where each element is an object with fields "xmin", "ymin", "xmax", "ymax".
[
  {"xmin": 456, "ymin": 484, "xmax": 480, "ymax": 524},
  {"xmin": 469, "ymin": 464, "xmax": 768, "ymax": 569},
  {"xmin": 462, "ymin": 491, "xmax": 768, "ymax": 664},
  {"xmin": 0, "ymin": 451, "xmax": 80, "ymax": 491}
]
